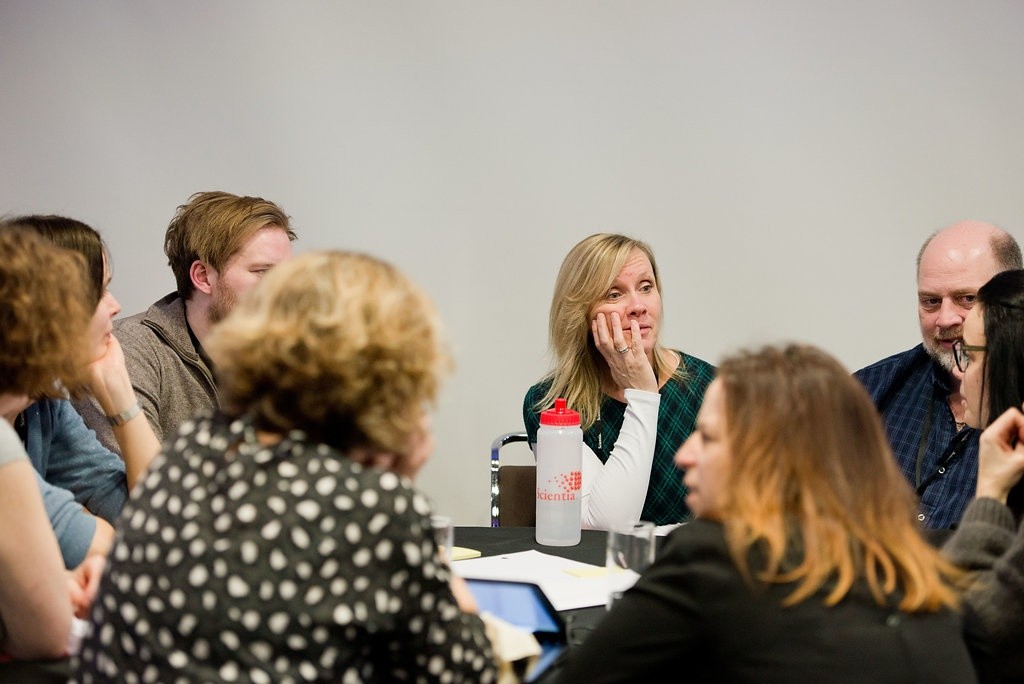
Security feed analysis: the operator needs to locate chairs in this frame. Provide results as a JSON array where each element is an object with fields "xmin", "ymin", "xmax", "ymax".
[{"xmin": 489, "ymin": 431, "xmax": 536, "ymax": 528}]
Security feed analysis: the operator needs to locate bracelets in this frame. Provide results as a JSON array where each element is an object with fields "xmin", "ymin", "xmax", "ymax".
[{"xmin": 105, "ymin": 400, "xmax": 148, "ymax": 426}]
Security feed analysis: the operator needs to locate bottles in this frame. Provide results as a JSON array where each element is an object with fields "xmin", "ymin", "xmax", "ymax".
[{"xmin": 535, "ymin": 398, "xmax": 583, "ymax": 547}]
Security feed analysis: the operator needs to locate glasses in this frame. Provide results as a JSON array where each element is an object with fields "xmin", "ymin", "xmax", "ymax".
[{"xmin": 952, "ymin": 340, "xmax": 989, "ymax": 373}]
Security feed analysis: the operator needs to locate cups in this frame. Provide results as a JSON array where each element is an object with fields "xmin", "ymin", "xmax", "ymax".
[
  {"xmin": 429, "ymin": 515, "xmax": 453, "ymax": 564},
  {"xmin": 604, "ymin": 521, "xmax": 656, "ymax": 610}
]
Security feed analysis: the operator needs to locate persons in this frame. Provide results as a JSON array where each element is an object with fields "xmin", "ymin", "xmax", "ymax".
[
  {"xmin": 69, "ymin": 248, "xmax": 506, "ymax": 684},
  {"xmin": 937, "ymin": 407, "xmax": 1024, "ymax": 684},
  {"xmin": 952, "ymin": 271, "xmax": 1024, "ymax": 431},
  {"xmin": 0, "ymin": 214, "xmax": 163, "ymax": 684},
  {"xmin": 522, "ymin": 233, "xmax": 719, "ymax": 545},
  {"xmin": 849, "ymin": 219, "xmax": 1021, "ymax": 533},
  {"xmin": 70, "ymin": 191, "xmax": 300, "ymax": 466},
  {"xmin": 549, "ymin": 345, "xmax": 978, "ymax": 684}
]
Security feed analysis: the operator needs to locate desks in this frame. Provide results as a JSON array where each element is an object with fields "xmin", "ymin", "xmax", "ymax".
[{"xmin": 452, "ymin": 527, "xmax": 666, "ymax": 684}]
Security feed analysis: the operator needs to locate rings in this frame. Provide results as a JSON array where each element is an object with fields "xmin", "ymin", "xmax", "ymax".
[{"xmin": 619, "ymin": 347, "xmax": 629, "ymax": 354}]
